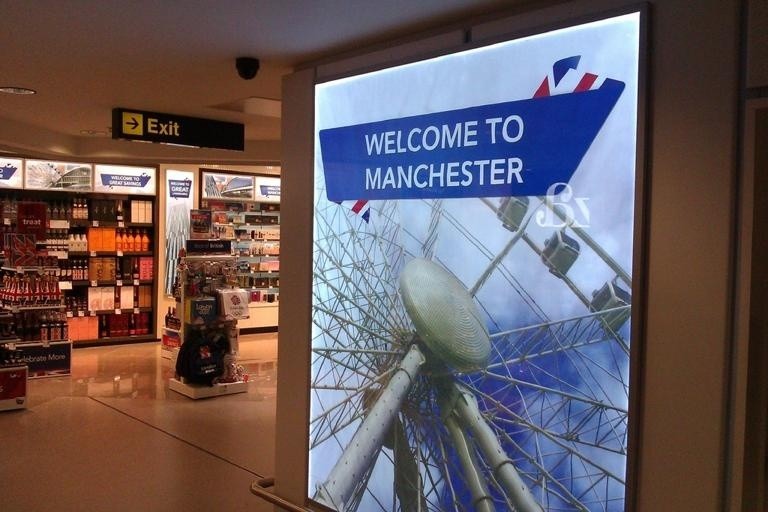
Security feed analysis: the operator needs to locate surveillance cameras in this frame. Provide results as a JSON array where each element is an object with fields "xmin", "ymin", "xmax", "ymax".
[{"xmin": 236, "ymin": 57, "xmax": 259, "ymax": 80}]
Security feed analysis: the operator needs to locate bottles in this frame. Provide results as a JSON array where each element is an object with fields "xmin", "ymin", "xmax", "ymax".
[
  {"xmin": 164, "ymin": 248, "xmax": 185, "ymax": 331},
  {"xmin": 230, "ymin": 242, "xmax": 279, "ymax": 257},
  {"xmin": 0, "ymin": 196, "xmax": 153, "ymax": 346},
  {"xmin": 190, "ymin": 225, "xmax": 227, "ymax": 256}
]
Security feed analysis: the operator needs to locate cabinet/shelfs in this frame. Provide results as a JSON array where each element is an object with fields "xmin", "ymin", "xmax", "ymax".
[
  {"xmin": 168, "ymin": 235, "xmax": 253, "ymax": 399},
  {"xmin": 0, "ymin": 259, "xmax": 72, "ymax": 375},
  {"xmin": 200, "ymin": 171, "xmax": 279, "ymax": 331},
  {"xmin": 0, "ymin": 193, "xmax": 157, "ymax": 348}
]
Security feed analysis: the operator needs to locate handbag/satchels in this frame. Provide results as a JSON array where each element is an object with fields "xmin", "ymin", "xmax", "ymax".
[
  {"xmin": 176, "ymin": 334, "xmax": 224, "ymax": 385},
  {"xmin": 214, "ymin": 288, "xmax": 250, "ymax": 321},
  {"xmin": 185, "ymin": 297, "xmax": 216, "ymax": 325}
]
